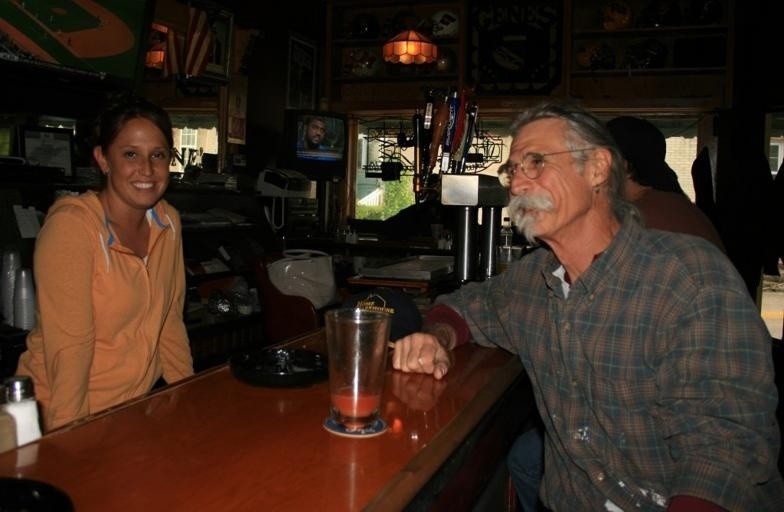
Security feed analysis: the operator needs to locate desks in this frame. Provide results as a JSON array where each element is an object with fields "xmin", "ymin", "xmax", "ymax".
[
  {"xmin": 0, "ymin": 310, "xmax": 526, "ymax": 510},
  {"xmin": 345, "ymin": 254, "xmax": 456, "ymax": 296}
]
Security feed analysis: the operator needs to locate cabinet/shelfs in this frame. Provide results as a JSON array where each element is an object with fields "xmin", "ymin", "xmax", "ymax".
[
  {"xmin": 319, "ymin": 1, "xmax": 464, "ymax": 115},
  {"xmin": 564, "ymin": 2, "xmax": 735, "ymax": 116},
  {"xmin": 0, "ymin": 178, "xmax": 263, "ymax": 377}
]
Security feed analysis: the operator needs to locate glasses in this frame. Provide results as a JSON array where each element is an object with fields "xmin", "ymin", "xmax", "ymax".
[{"xmin": 498, "ymin": 147, "xmax": 595, "ymax": 188}]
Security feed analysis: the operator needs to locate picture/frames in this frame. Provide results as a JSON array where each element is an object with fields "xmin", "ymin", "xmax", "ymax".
[{"xmin": 14, "ymin": 126, "xmax": 78, "ymax": 181}]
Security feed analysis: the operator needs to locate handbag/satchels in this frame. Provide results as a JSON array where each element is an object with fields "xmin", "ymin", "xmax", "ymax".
[{"xmin": 266, "ymin": 249, "xmax": 335, "ymax": 309}]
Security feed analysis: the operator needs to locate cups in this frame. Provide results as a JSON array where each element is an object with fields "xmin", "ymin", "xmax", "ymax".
[
  {"xmin": 324, "ymin": 309, "xmax": 392, "ymax": 430},
  {"xmin": 1, "ymin": 250, "xmax": 37, "ymax": 330}
]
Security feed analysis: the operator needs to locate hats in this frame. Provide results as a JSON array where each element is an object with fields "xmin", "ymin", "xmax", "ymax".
[{"xmin": 607, "ymin": 116, "xmax": 678, "ymax": 185}]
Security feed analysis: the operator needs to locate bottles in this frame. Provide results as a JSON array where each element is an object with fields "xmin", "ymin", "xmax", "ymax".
[{"xmin": 2, "ymin": 375, "xmax": 43, "ymax": 446}]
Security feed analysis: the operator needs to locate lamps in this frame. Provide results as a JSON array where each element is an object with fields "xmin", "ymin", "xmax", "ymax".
[{"xmin": 379, "ymin": 28, "xmax": 438, "ymax": 66}]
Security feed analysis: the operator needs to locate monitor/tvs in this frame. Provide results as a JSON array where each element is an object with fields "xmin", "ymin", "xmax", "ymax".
[{"xmin": 283, "ymin": 108, "xmax": 349, "ymax": 181}]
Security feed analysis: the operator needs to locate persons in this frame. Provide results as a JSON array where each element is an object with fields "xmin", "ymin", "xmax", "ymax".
[
  {"xmin": 297, "ymin": 116, "xmax": 329, "ymax": 151},
  {"xmin": 393, "ymin": 99, "xmax": 783, "ymax": 510},
  {"xmin": 16, "ymin": 101, "xmax": 198, "ymax": 439},
  {"xmin": 598, "ymin": 111, "xmax": 731, "ymax": 262}
]
